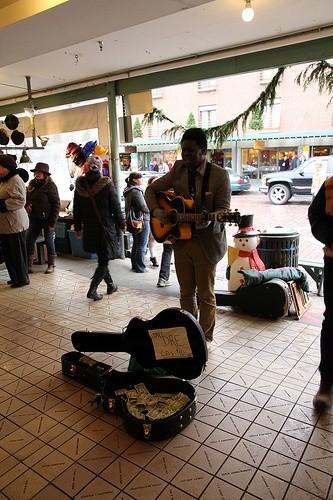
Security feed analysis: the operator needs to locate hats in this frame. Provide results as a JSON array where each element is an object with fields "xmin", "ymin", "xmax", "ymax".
[
  {"xmin": 30, "ymin": 162, "xmax": 51, "ymax": 176},
  {"xmin": 65, "ymin": 140, "xmax": 108, "ymax": 165},
  {"xmin": 0, "ymin": 155, "xmax": 17, "ymax": 170},
  {"xmin": 86, "ymin": 155, "xmax": 103, "ymax": 173}
]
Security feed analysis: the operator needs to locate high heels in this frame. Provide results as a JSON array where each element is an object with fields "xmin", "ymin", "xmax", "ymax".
[{"xmin": 150, "ymin": 256, "xmax": 159, "ymax": 267}]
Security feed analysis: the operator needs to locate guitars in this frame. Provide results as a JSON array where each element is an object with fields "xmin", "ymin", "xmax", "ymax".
[{"xmin": 150, "ymin": 191, "xmax": 241, "ymax": 245}]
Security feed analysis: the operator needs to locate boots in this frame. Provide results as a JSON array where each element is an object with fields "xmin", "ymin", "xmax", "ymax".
[
  {"xmin": 46, "ymin": 254, "xmax": 57, "ymax": 273},
  {"xmin": 27, "ymin": 255, "xmax": 35, "ymax": 273},
  {"xmin": 103, "ymin": 269, "xmax": 118, "ymax": 294},
  {"xmin": 87, "ymin": 267, "xmax": 103, "ymax": 299}
]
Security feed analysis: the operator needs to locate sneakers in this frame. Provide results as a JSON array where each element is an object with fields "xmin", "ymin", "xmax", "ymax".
[{"xmin": 157, "ymin": 278, "xmax": 173, "ymax": 287}]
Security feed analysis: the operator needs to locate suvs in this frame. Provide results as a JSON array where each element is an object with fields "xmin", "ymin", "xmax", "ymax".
[{"xmin": 258, "ymin": 154, "xmax": 333, "ymax": 206}]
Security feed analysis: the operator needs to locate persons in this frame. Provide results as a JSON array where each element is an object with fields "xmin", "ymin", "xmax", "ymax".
[
  {"xmin": 121, "ymin": 171, "xmax": 149, "ymax": 274},
  {"xmin": 145, "ymin": 175, "xmax": 160, "ymax": 267},
  {"xmin": 72, "ymin": 155, "xmax": 125, "ymax": 302},
  {"xmin": 0, "ymin": 155, "xmax": 30, "ymax": 288},
  {"xmin": 24, "ymin": 162, "xmax": 60, "ymax": 273},
  {"xmin": 277, "ymin": 153, "xmax": 305, "ymax": 172},
  {"xmin": 307, "ymin": 174, "xmax": 333, "ymax": 411},
  {"xmin": 144, "ymin": 129, "xmax": 231, "ymax": 347},
  {"xmin": 135, "ymin": 158, "xmax": 172, "ymax": 173}
]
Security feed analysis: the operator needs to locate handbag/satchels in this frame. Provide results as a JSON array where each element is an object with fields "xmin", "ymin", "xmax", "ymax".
[
  {"xmin": 126, "ymin": 206, "xmax": 143, "ymax": 234},
  {"xmin": 97, "ymin": 222, "xmax": 126, "ymax": 260}
]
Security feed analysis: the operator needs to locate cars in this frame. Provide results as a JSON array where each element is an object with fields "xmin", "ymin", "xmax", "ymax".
[{"xmin": 225, "ymin": 169, "xmax": 251, "ymax": 195}]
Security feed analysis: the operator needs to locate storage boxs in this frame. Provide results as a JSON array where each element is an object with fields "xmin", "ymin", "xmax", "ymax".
[
  {"xmin": 67, "ymin": 230, "xmax": 98, "ymax": 259},
  {"xmin": 258, "ymin": 225, "xmax": 301, "ymax": 270}
]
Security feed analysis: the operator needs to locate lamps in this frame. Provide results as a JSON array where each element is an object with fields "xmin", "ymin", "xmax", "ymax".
[{"xmin": 241, "ymin": 0, "xmax": 254, "ymax": 22}]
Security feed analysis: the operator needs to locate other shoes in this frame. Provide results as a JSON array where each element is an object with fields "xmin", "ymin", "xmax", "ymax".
[
  {"xmin": 7, "ymin": 280, "xmax": 13, "ymax": 284},
  {"xmin": 312, "ymin": 388, "xmax": 333, "ymax": 409},
  {"xmin": 10, "ymin": 281, "xmax": 30, "ymax": 288}
]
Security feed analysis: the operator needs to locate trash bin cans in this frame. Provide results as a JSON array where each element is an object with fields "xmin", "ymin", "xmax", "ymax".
[{"xmin": 257, "ymin": 225, "xmax": 299, "ymax": 268}]
